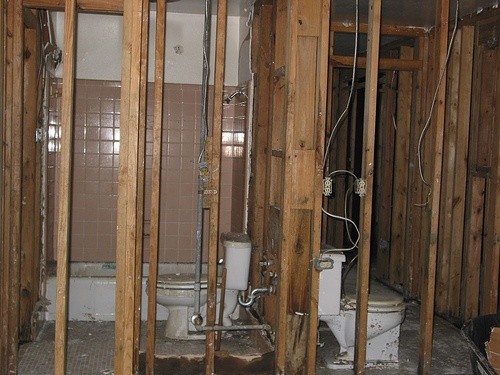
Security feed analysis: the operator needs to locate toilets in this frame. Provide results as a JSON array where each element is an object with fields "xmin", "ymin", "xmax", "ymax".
[
  {"xmin": 318, "ymin": 241, "xmax": 406, "ymax": 365},
  {"xmin": 145, "ymin": 234, "xmax": 253, "ymax": 342}
]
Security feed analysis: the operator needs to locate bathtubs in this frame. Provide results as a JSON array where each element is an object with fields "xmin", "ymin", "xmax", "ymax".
[{"xmin": 41, "ymin": 263, "xmax": 226, "ymax": 321}]
